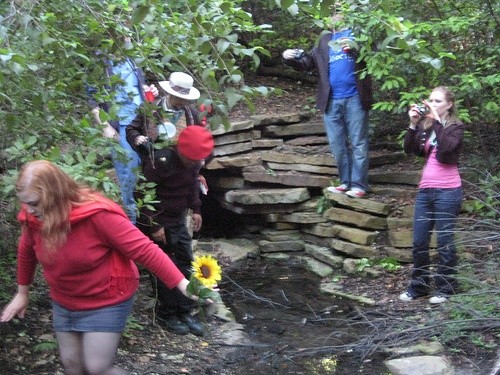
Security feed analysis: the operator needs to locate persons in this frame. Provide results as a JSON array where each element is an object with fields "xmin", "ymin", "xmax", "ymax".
[
  {"xmin": 135, "ymin": 125, "xmax": 214, "ymax": 337},
  {"xmin": 282, "ymin": 3, "xmax": 373, "ymax": 198},
  {"xmin": 125, "ymin": 71, "xmax": 215, "ymax": 262},
  {"xmin": 86, "ymin": 22, "xmax": 160, "ymax": 225},
  {"xmin": 1, "ymin": 159, "xmax": 213, "ymax": 375},
  {"xmin": 399, "ymin": 86, "xmax": 465, "ymax": 304}
]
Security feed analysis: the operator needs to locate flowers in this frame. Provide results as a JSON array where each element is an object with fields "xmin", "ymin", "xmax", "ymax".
[{"xmin": 187, "ymin": 255, "xmax": 219, "ymax": 348}]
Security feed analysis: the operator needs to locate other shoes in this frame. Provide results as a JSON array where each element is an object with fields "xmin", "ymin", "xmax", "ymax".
[
  {"xmin": 156, "ymin": 314, "xmax": 190, "ymax": 334},
  {"xmin": 176, "ymin": 311, "xmax": 204, "ymax": 336}
]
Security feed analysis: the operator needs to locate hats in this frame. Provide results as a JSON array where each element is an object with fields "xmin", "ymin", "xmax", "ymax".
[
  {"xmin": 177, "ymin": 125, "xmax": 215, "ymax": 160},
  {"xmin": 158, "ymin": 71, "xmax": 201, "ymax": 99}
]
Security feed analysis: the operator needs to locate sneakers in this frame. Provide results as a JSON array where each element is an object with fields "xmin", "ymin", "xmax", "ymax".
[
  {"xmin": 399, "ymin": 289, "xmax": 428, "ymax": 300},
  {"xmin": 327, "ymin": 183, "xmax": 348, "ymax": 193},
  {"xmin": 346, "ymin": 188, "xmax": 369, "ymax": 197},
  {"xmin": 429, "ymin": 295, "xmax": 450, "ymax": 303}
]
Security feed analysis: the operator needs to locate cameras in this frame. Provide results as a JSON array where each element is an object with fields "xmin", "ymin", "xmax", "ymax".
[
  {"xmin": 416, "ymin": 106, "xmax": 426, "ymax": 114},
  {"xmin": 294, "ymin": 49, "xmax": 305, "ymax": 59}
]
